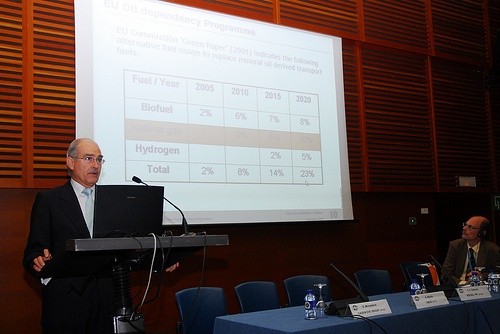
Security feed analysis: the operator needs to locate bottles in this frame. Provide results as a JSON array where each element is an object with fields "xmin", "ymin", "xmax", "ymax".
[
  {"xmin": 304, "ymin": 289, "xmax": 317, "ymax": 320},
  {"xmin": 469, "ymin": 271, "xmax": 479, "ymax": 287},
  {"xmin": 410, "ymin": 280, "xmax": 421, "ymax": 305}
]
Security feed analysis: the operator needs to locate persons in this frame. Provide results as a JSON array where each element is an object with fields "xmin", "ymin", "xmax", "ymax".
[
  {"xmin": 22, "ymin": 137, "xmax": 180, "ymax": 334},
  {"xmin": 443, "ymin": 217, "xmax": 500, "ymax": 290}
]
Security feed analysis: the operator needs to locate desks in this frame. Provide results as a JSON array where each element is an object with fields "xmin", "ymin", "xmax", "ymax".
[{"xmin": 214, "ymin": 290, "xmax": 500, "ymax": 334}]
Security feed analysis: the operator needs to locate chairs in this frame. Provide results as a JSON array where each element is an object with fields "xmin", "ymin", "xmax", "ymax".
[
  {"xmin": 398, "ymin": 260, "xmax": 437, "ymax": 291},
  {"xmin": 234, "ymin": 281, "xmax": 278, "ymax": 313},
  {"xmin": 283, "ymin": 275, "xmax": 332, "ymax": 307},
  {"xmin": 354, "ymin": 269, "xmax": 392, "ymax": 296},
  {"xmin": 175, "ymin": 287, "xmax": 225, "ymax": 334}
]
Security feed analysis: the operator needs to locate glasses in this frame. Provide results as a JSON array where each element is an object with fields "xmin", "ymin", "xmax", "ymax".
[
  {"xmin": 69, "ymin": 155, "xmax": 105, "ymax": 164},
  {"xmin": 463, "ymin": 222, "xmax": 483, "ymax": 230}
]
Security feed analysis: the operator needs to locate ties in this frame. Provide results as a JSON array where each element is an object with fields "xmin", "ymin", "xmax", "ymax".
[
  {"xmin": 465, "ymin": 248, "xmax": 477, "ymax": 286},
  {"xmin": 82, "ymin": 188, "xmax": 94, "ymax": 238}
]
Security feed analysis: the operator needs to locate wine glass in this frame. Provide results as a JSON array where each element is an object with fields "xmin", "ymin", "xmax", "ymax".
[
  {"xmin": 314, "ymin": 283, "xmax": 328, "ymax": 319},
  {"xmin": 417, "ymin": 274, "xmax": 429, "ymax": 294},
  {"xmin": 475, "ymin": 267, "xmax": 485, "ymax": 281}
]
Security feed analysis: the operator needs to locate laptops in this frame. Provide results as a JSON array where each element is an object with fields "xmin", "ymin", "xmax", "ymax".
[{"xmin": 94, "ymin": 185, "xmax": 164, "ymax": 238}]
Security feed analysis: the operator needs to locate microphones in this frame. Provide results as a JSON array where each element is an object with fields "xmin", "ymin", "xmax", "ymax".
[
  {"xmin": 132, "ymin": 176, "xmax": 189, "ymax": 235},
  {"xmin": 329, "ymin": 264, "xmax": 371, "ymax": 302},
  {"xmin": 429, "ymin": 255, "xmax": 458, "ymax": 288}
]
